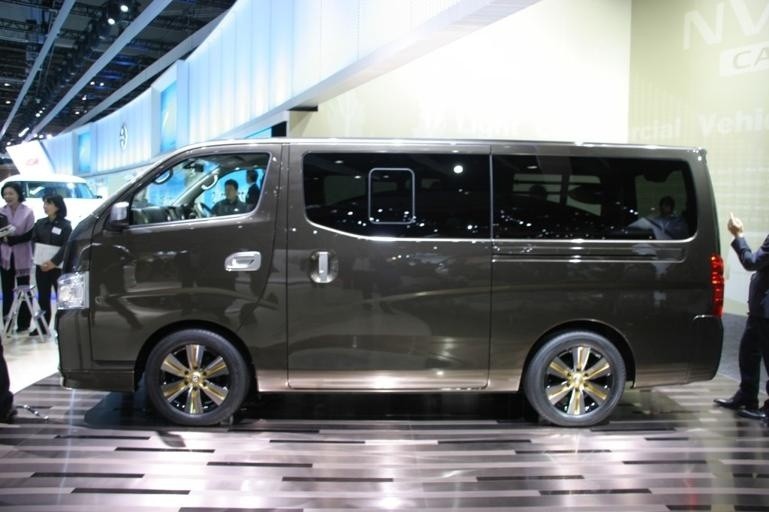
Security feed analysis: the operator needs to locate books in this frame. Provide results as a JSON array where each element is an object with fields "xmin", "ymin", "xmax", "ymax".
[{"xmin": 34, "ymin": 242, "xmax": 64, "ymax": 270}]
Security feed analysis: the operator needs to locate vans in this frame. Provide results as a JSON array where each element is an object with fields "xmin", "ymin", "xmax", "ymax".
[
  {"xmin": 1, "ymin": 171, "xmax": 106, "ymax": 233},
  {"xmin": 54, "ymin": 136, "xmax": 729, "ymax": 431}
]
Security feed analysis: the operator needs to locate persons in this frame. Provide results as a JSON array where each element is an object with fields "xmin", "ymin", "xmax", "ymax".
[
  {"xmin": 210, "ymin": 180, "xmax": 247, "ymax": 216},
  {"xmin": 1, "ymin": 348, "xmax": 18, "ymax": 424},
  {"xmin": 713, "ymin": 217, "xmax": 769, "ymax": 420},
  {"xmin": 0, "ymin": 182, "xmax": 35, "ymax": 333},
  {"xmin": 648, "ymin": 196, "xmax": 688, "ymax": 240},
  {"xmin": 245, "ymin": 170, "xmax": 260, "ymax": 211},
  {"xmin": 29, "ymin": 194, "xmax": 72, "ymax": 336}
]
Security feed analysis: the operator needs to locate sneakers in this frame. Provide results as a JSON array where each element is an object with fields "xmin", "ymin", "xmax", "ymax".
[{"xmin": 15, "ymin": 324, "xmax": 50, "ymax": 338}]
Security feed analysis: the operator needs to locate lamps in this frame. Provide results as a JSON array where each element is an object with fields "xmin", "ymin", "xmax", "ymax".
[{"xmin": 31, "ymin": 0, "xmax": 134, "ymax": 120}]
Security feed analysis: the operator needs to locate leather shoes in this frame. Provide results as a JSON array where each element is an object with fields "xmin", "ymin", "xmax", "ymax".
[
  {"xmin": 735, "ymin": 405, "xmax": 769, "ymax": 422},
  {"xmin": 711, "ymin": 390, "xmax": 760, "ymax": 410}
]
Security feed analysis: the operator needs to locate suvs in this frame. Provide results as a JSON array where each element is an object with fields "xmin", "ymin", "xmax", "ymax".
[{"xmin": 338, "ymin": 186, "xmax": 678, "ymax": 252}]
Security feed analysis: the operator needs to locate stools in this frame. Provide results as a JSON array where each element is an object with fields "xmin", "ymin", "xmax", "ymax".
[{"xmin": 5, "ymin": 284, "xmax": 50, "ymax": 343}]
